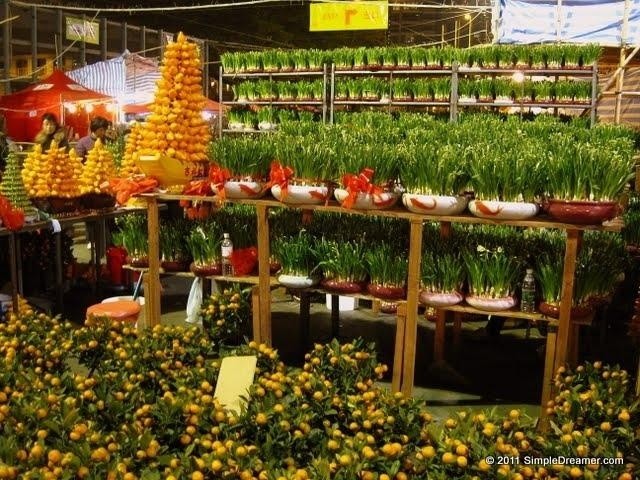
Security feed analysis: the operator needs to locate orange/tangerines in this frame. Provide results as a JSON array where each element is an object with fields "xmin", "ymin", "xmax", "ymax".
[{"xmin": 0, "ymin": 288, "xmax": 635, "ymax": 480}]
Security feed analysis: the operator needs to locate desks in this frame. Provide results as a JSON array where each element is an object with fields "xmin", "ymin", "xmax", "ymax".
[{"xmin": 0, "ymin": 202, "xmax": 166, "ymax": 314}]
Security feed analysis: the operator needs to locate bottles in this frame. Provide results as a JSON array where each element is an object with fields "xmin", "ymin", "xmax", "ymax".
[
  {"xmin": 220, "ymin": 232, "xmax": 234, "ymax": 279},
  {"xmin": 519, "ymin": 268, "xmax": 537, "ymax": 315}
]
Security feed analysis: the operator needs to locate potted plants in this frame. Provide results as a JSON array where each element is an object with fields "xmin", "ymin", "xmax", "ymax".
[{"xmin": 121, "ymin": 62, "xmax": 622, "ymax": 434}]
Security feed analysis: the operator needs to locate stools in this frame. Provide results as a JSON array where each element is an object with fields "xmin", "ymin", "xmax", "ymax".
[{"xmin": 85, "ymin": 300, "xmax": 142, "ymax": 332}]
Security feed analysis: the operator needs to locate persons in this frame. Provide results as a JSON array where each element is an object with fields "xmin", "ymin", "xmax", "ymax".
[
  {"xmin": 0, "ymin": 113, "xmax": 18, "ymax": 177},
  {"xmin": 73, "ymin": 116, "xmax": 118, "ymax": 251},
  {"xmin": 23, "ymin": 112, "xmax": 68, "ymax": 156}
]
